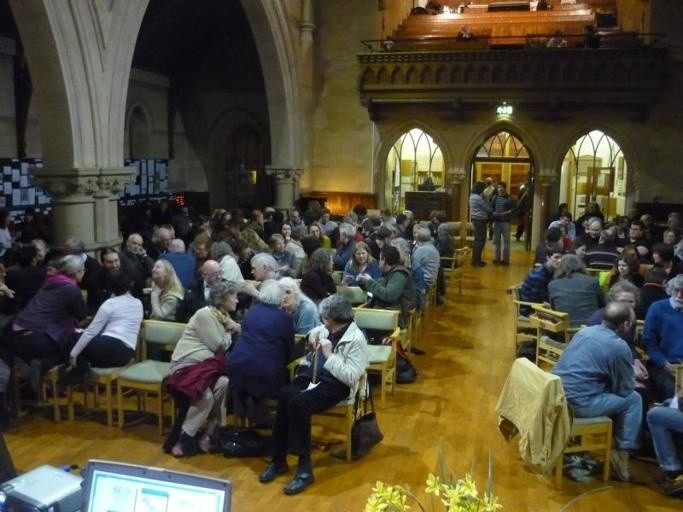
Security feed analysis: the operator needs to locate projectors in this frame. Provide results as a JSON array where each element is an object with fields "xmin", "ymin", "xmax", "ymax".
[{"xmin": 0, "ymin": 464, "xmax": 84, "ymax": 512}]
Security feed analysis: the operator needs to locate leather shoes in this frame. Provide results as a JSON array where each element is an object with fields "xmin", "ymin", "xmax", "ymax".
[
  {"xmin": 283, "ymin": 473, "xmax": 316, "ymax": 494},
  {"xmin": 259, "ymin": 460, "xmax": 290, "ymax": 483}
]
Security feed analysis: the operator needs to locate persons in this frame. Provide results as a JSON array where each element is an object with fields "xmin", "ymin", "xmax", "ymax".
[
  {"xmin": 1, "ymin": 198, "xmax": 457, "ymax": 493},
  {"xmin": 468, "ymin": 180, "xmax": 496, "ymax": 267},
  {"xmin": 519, "ymin": 200, "xmax": 683, "ymax": 498},
  {"xmin": 489, "ymin": 181, "xmax": 517, "ymax": 266},
  {"xmin": 482, "ymin": 177, "xmax": 497, "ymax": 241},
  {"xmin": 511, "ymin": 185, "xmax": 531, "ymax": 242}
]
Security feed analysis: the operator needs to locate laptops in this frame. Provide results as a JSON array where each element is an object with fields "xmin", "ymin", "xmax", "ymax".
[{"xmin": 81, "ymin": 459, "xmax": 231, "ymax": 512}]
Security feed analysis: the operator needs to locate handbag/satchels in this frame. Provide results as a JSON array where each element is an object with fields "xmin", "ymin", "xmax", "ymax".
[
  {"xmin": 560, "ymin": 441, "xmax": 604, "ymax": 483},
  {"xmin": 221, "ymin": 425, "xmax": 264, "ymax": 458},
  {"xmin": 397, "ymin": 352, "xmax": 417, "ymax": 383},
  {"xmin": 352, "ymin": 412, "xmax": 384, "ymax": 460}
]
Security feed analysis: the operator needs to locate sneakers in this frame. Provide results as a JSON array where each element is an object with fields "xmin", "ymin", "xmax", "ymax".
[
  {"xmin": 656, "ymin": 474, "xmax": 683, "ymax": 495},
  {"xmin": 471, "ymin": 259, "xmax": 511, "ymax": 268},
  {"xmin": 70, "ymin": 370, "xmax": 96, "ymax": 388},
  {"xmin": 199, "ymin": 430, "xmax": 217, "ymax": 452},
  {"xmin": 172, "ymin": 432, "xmax": 195, "ymax": 457},
  {"xmin": 608, "ymin": 448, "xmax": 633, "ymax": 483}
]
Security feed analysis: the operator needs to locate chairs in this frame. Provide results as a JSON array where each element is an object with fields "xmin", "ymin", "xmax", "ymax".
[{"xmin": 508, "ymin": 269, "xmax": 683, "ymax": 491}]
